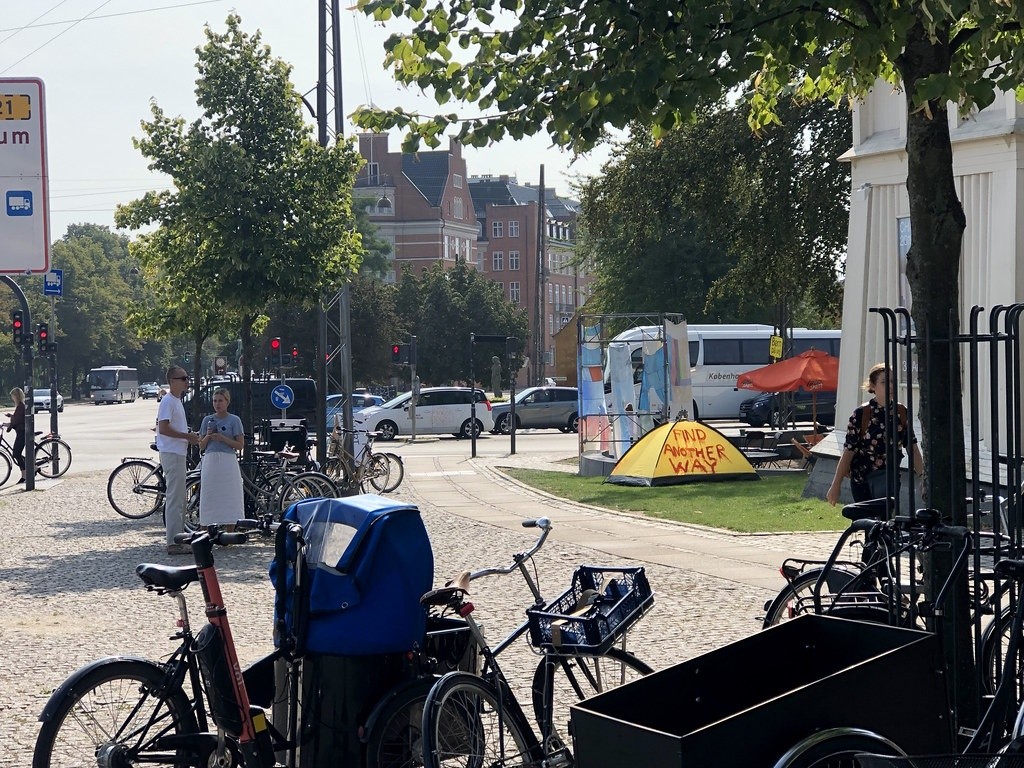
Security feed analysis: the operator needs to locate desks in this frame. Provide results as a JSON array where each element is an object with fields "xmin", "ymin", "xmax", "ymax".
[
  {"xmin": 743, "ymin": 452, "xmax": 780, "ymax": 469},
  {"xmin": 725, "ymin": 433, "xmax": 747, "ymax": 451}
]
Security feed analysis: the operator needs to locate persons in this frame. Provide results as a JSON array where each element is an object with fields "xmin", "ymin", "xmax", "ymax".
[
  {"xmin": 156, "ymin": 365, "xmax": 202, "ymax": 555},
  {"xmin": 198, "ymin": 389, "xmax": 245, "ymax": 533},
  {"xmin": 534, "ymin": 392, "xmax": 542, "ymax": 403},
  {"xmin": 491, "ymin": 356, "xmax": 503, "ymax": 399},
  {"xmin": 151, "ymin": 390, "xmax": 166, "ymax": 432},
  {"xmin": 826, "ymin": 363, "xmax": 925, "ymax": 580},
  {"xmin": 3, "ymin": 387, "xmax": 41, "ymax": 484},
  {"xmin": 417, "ymin": 396, "xmax": 428, "ymax": 406},
  {"xmin": 547, "ymin": 392, "xmax": 556, "ymax": 403}
]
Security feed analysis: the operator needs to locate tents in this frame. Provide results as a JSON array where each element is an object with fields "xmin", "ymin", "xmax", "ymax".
[{"xmin": 601, "ymin": 418, "xmax": 761, "ymax": 488}]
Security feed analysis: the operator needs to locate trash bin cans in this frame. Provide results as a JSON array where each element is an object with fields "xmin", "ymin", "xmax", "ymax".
[{"xmin": 262, "ymin": 418, "xmax": 307, "ymax": 488}]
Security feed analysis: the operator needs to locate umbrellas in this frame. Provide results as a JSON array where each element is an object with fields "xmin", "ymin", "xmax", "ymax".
[{"xmin": 737, "ymin": 347, "xmax": 839, "ymax": 445}]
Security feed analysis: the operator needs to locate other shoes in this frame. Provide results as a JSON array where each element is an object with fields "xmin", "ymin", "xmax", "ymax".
[{"xmin": 167, "ymin": 544, "xmax": 193, "ymax": 555}]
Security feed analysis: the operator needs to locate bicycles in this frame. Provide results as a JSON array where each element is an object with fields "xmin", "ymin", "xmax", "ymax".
[
  {"xmin": 756, "ymin": 481, "xmax": 1024, "ymax": 704},
  {"xmin": 108, "ymin": 425, "xmax": 404, "ymax": 534},
  {"xmin": 33, "ymin": 492, "xmax": 658, "ymax": 768},
  {"xmin": 0, "ymin": 424, "xmax": 72, "ymax": 486}
]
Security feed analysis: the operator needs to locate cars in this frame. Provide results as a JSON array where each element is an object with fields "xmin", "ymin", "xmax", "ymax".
[
  {"xmin": 33, "ymin": 389, "xmax": 64, "ymax": 414},
  {"xmin": 139, "ymin": 382, "xmax": 171, "ymax": 400}
]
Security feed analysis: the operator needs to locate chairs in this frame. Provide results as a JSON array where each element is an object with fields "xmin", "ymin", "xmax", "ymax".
[{"xmin": 746, "ymin": 431, "xmax": 823, "ymax": 469}]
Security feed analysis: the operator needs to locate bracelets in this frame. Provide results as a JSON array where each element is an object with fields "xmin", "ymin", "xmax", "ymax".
[{"xmin": 918, "ymin": 474, "xmax": 922, "ymax": 477}]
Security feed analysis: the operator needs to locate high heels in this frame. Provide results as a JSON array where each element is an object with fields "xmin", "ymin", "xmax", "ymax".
[{"xmin": 16, "ymin": 467, "xmax": 41, "ymax": 485}]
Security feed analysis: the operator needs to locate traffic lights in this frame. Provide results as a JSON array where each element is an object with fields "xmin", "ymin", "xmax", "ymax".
[
  {"xmin": 13, "ymin": 310, "xmax": 23, "ymax": 343},
  {"xmin": 38, "ymin": 323, "xmax": 48, "ymax": 353},
  {"xmin": 271, "ymin": 337, "xmax": 282, "ymax": 364},
  {"xmin": 326, "ymin": 345, "xmax": 332, "ymax": 369},
  {"xmin": 184, "ymin": 352, "xmax": 190, "ymax": 362},
  {"xmin": 391, "ymin": 345, "xmax": 399, "ymax": 361}
]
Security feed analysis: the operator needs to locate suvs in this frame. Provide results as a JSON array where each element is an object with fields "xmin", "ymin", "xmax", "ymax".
[
  {"xmin": 487, "ymin": 387, "xmax": 579, "ymax": 435},
  {"xmin": 324, "ymin": 394, "xmax": 385, "ymax": 432}
]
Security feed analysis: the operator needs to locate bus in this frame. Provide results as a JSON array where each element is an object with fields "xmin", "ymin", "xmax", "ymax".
[
  {"xmin": 602, "ymin": 323, "xmax": 842, "ymax": 429},
  {"xmin": 86, "ymin": 365, "xmax": 139, "ymax": 405}
]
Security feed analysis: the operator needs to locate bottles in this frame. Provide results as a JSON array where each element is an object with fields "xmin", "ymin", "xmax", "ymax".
[{"xmin": 207, "ymin": 415, "xmax": 218, "ymax": 433}]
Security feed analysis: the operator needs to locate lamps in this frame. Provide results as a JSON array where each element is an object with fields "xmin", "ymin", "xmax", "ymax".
[{"xmin": 857, "ymin": 182, "xmax": 872, "ymax": 201}]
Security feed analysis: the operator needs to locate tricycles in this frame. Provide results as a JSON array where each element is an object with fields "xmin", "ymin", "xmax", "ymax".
[{"xmin": 568, "ymin": 502, "xmax": 1024, "ymax": 768}]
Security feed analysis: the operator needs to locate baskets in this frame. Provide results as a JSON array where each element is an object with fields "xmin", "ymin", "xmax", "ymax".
[{"xmin": 524, "ymin": 565, "xmax": 657, "ymax": 659}]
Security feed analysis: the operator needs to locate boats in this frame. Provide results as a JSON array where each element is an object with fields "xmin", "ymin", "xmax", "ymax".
[{"xmin": 292, "ymin": 346, "xmax": 298, "ymax": 362}]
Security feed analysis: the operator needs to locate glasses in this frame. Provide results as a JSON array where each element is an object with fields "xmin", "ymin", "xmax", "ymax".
[{"xmin": 171, "ymin": 375, "xmax": 190, "ymax": 381}]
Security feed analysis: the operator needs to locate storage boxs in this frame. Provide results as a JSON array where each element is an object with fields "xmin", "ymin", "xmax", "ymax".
[{"xmin": 525, "ymin": 565, "xmax": 654, "ymax": 660}]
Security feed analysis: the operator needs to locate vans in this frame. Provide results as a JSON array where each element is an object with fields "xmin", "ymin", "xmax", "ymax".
[
  {"xmin": 356, "ymin": 385, "xmax": 495, "ymax": 440},
  {"xmin": 740, "ymin": 386, "xmax": 839, "ymax": 428}
]
